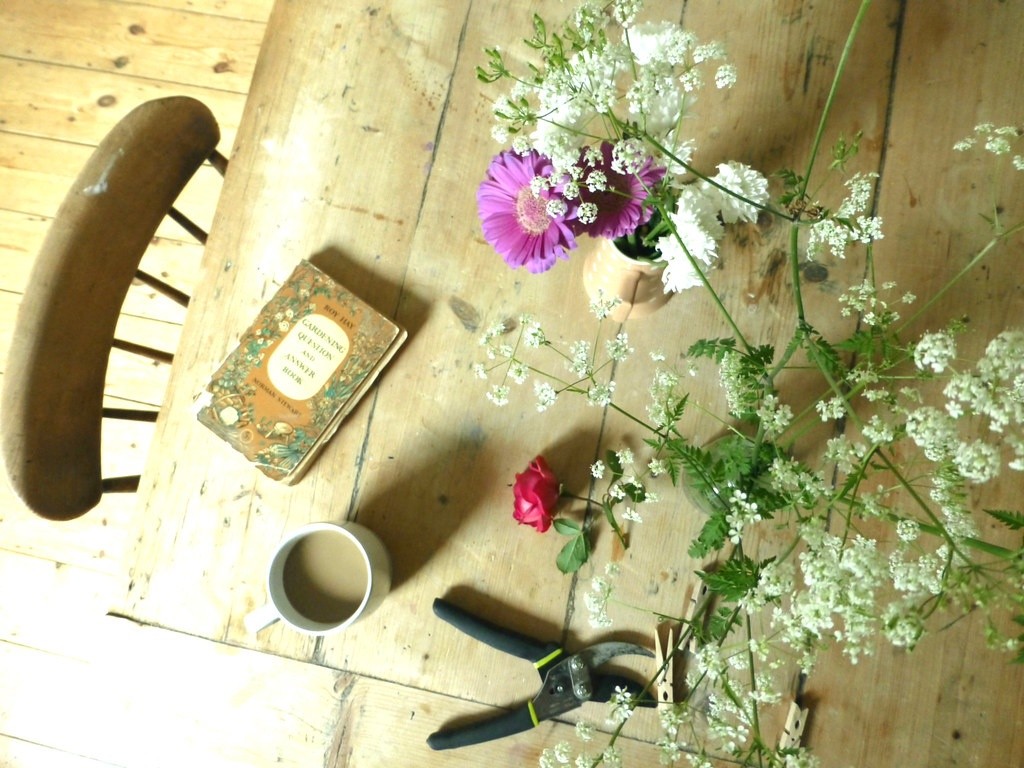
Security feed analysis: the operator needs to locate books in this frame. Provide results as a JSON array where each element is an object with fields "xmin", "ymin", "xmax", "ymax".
[{"xmin": 186, "ymin": 259, "xmax": 408, "ymax": 487}]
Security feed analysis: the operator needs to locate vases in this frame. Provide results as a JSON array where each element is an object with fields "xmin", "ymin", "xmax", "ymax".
[{"xmin": 583, "ymin": 236, "xmax": 675, "ymax": 322}]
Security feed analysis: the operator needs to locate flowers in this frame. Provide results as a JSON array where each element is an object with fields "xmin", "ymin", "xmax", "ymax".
[
  {"xmin": 513, "ymin": 450, "xmax": 646, "ymax": 576},
  {"xmin": 473, "ymin": 0, "xmax": 771, "ymax": 294},
  {"xmin": 478, "ymin": 0, "xmax": 1024, "ymax": 768}
]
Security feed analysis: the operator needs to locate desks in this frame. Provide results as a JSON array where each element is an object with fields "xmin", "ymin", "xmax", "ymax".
[{"xmin": 101, "ymin": 0, "xmax": 1024, "ymax": 768}]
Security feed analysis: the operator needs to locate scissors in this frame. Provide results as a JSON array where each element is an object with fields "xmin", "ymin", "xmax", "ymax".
[{"xmin": 426, "ymin": 595, "xmax": 663, "ymax": 752}]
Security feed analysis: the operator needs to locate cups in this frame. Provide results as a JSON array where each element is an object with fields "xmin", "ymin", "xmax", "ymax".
[{"xmin": 266, "ymin": 521, "xmax": 395, "ymax": 636}]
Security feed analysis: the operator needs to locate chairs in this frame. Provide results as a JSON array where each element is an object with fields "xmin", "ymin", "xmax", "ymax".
[{"xmin": 0, "ymin": 95, "xmax": 228, "ymax": 521}]
